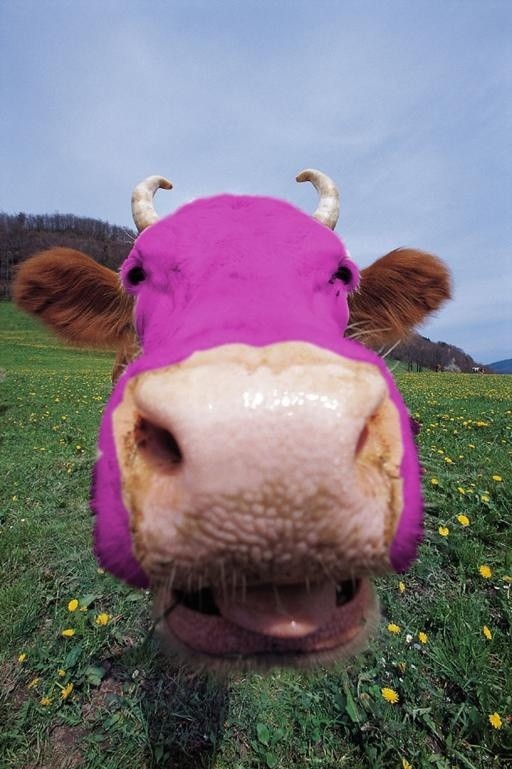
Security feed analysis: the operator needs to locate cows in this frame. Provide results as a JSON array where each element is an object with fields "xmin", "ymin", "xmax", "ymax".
[{"xmin": 11, "ymin": 168, "xmax": 453, "ymax": 687}]
